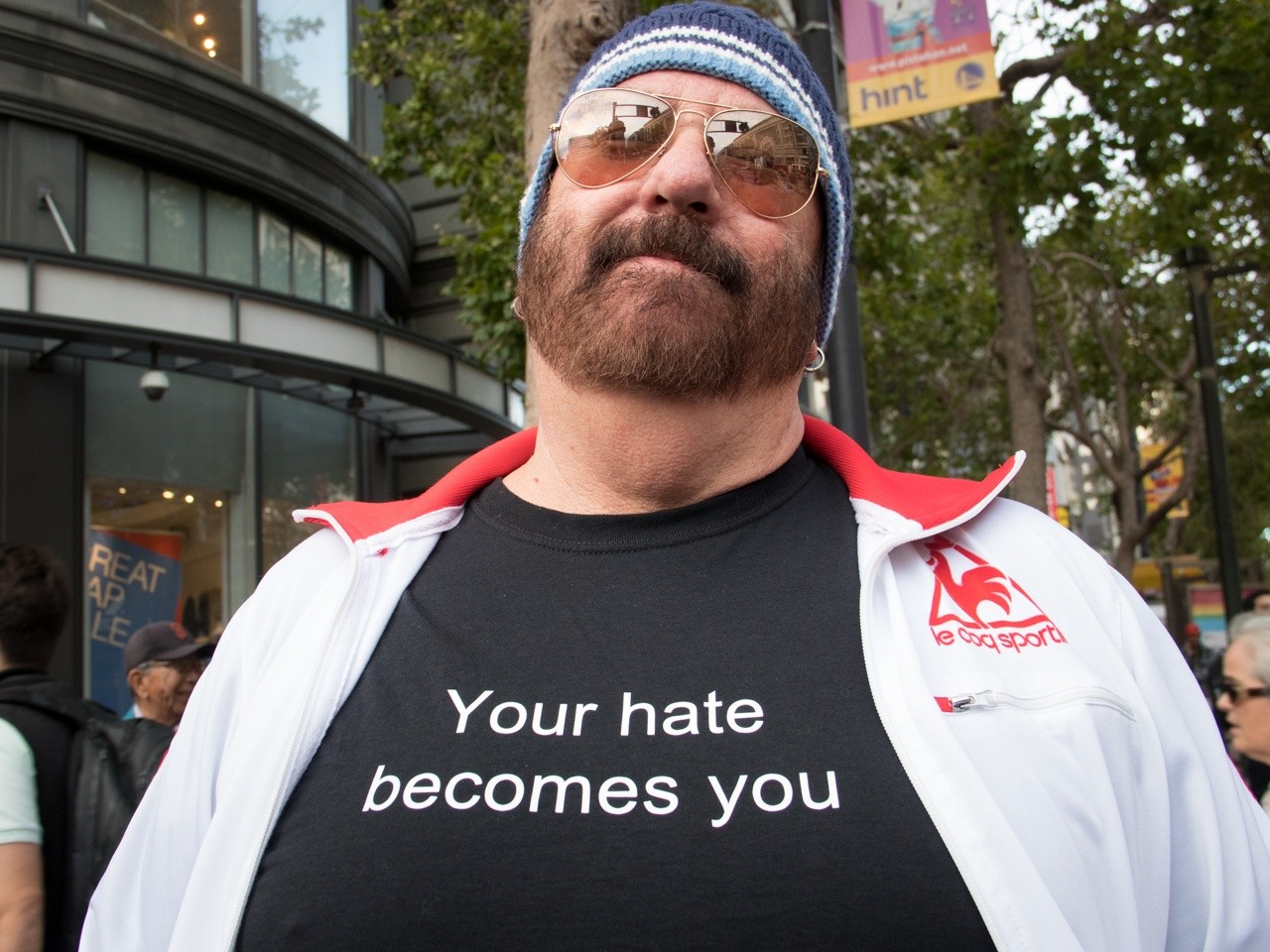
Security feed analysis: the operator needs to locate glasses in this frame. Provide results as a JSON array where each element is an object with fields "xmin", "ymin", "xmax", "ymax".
[
  {"xmin": 1209, "ymin": 675, "xmax": 1270, "ymax": 705},
  {"xmin": 546, "ymin": 87, "xmax": 832, "ymax": 221}
]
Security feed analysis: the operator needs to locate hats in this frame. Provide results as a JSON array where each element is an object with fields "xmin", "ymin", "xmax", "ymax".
[
  {"xmin": 122, "ymin": 622, "xmax": 216, "ymax": 676},
  {"xmin": 517, "ymin": 0, "xmax": 850, "ymax": 350},
  {"xmin": 1184, "ymin": 624, "xmax": 1200, "ymax": 636}
]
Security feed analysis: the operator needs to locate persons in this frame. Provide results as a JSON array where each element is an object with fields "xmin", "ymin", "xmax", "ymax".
[
  {"xmin": 1, "ymin": 540, "xmax": 1270, "ymax": 952},
  {"xmin": 78, "ymin": 0, "xmax": 1269, "ymax": 952}
]
